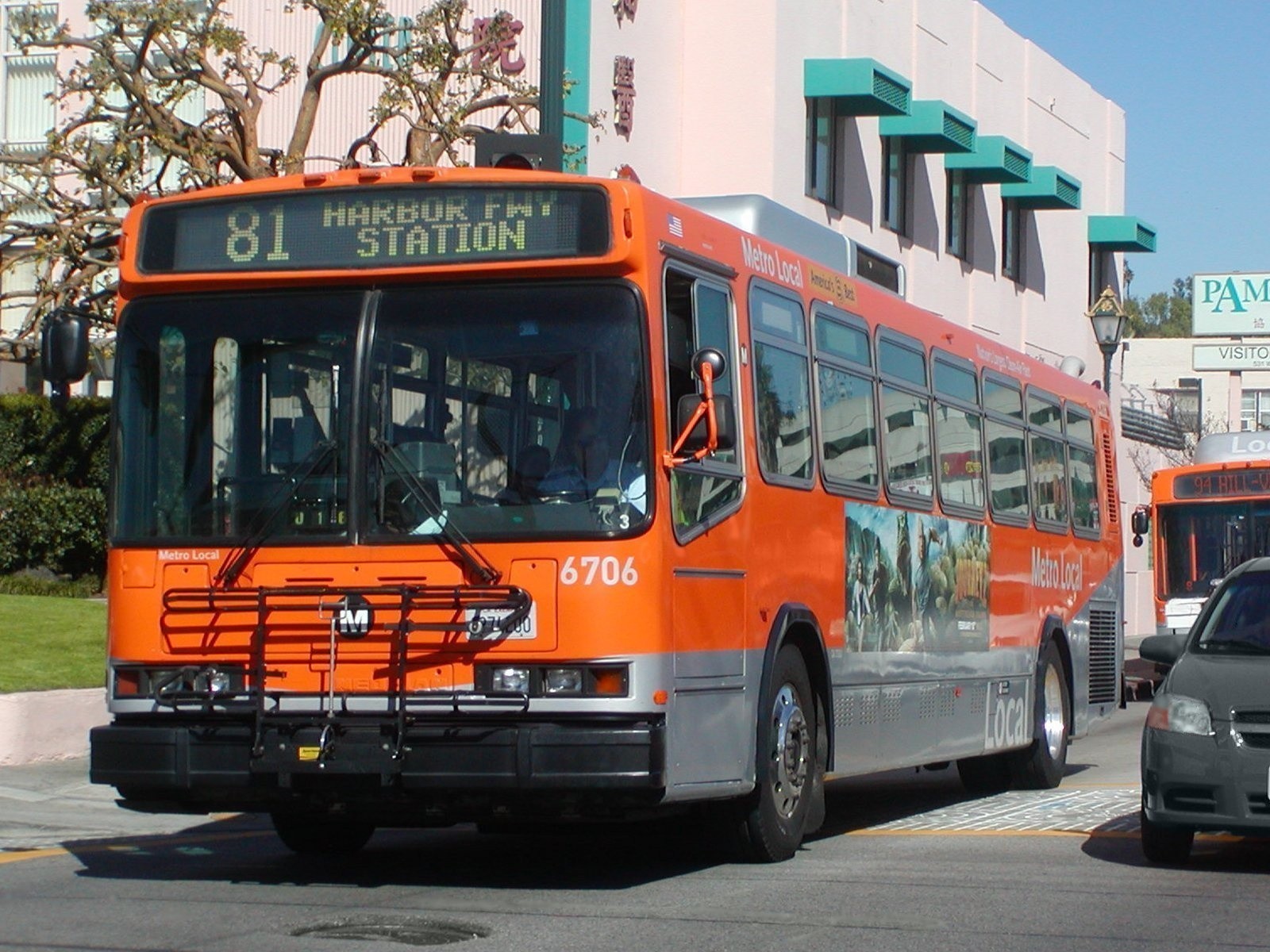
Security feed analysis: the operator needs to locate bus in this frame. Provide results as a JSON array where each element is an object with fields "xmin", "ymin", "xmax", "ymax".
[
  {"xmin": 86, "ymin": 163, "xmax": 1127, "ymax": 862},
  {"xmin": 1131, "ymin": 428, "xmax": 1270, "ymax": 634}
]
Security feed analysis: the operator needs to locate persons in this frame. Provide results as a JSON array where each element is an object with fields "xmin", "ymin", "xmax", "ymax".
[{"xmin": 494, "ymin": 416, "xmax": 649, "ymax": 513}]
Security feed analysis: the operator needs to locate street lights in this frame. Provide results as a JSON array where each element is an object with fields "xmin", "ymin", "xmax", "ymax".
[{"xmin": 1084, "ymin": 282, "xmax": 1128, "ymax": 403}]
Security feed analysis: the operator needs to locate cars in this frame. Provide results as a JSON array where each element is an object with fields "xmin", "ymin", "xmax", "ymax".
[{"xmin": 1138, "ymin": 556, "xmax": 1270, "ymax": 862}]
